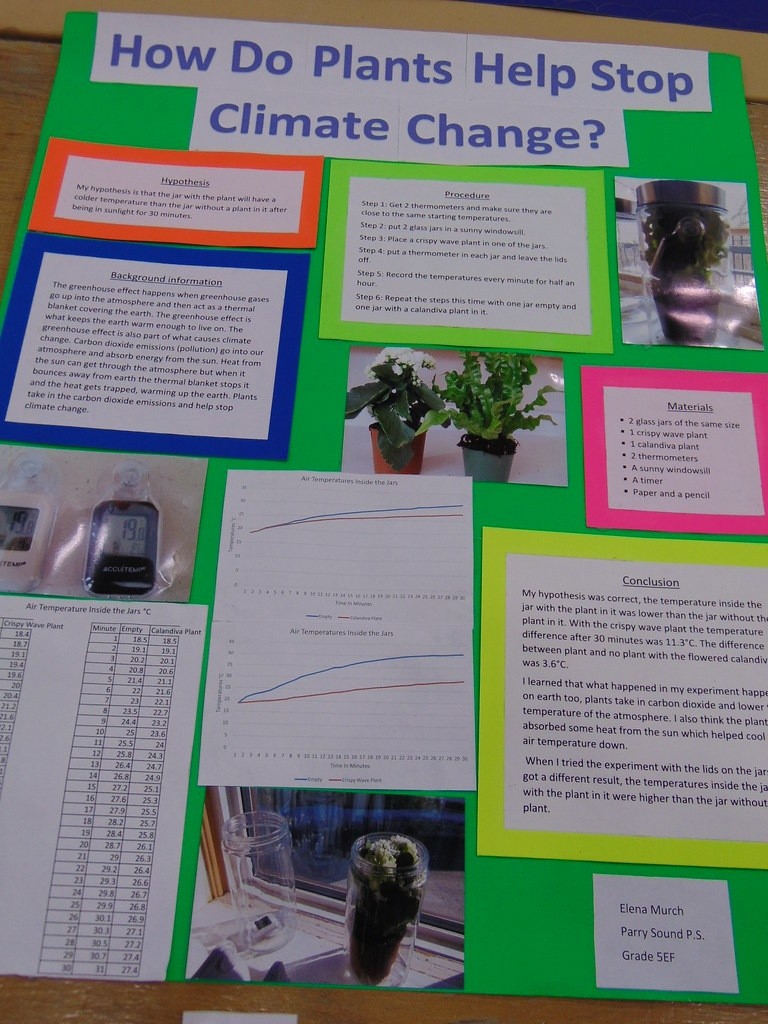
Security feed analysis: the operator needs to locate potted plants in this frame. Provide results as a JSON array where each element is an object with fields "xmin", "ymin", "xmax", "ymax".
[{"xmin": 415, "ymin": 351, "xmax": 564, "ymax": 483}]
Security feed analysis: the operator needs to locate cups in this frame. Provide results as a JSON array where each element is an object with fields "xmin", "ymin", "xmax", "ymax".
[
  {"xmin": 220, "ymin": 812, "xmax": 298, "ymax": 954},
  {"xmin": 634, "ymin": 181, "xmax": 731, "ymax": 347},
  {"xmin": 347, "ymin": 832, "xmax": 430, "ymax": 989}
]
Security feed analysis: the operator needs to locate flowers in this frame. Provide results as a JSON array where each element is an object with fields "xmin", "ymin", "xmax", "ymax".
[{"xmin": 346, "ymin": 347, "xmax": 450, "ymax": 471}]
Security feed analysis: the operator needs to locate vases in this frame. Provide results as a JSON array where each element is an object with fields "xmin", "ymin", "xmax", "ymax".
[{"xmin": 369, "ymin": 420, "xmax": 426, "ymax": 475}]
